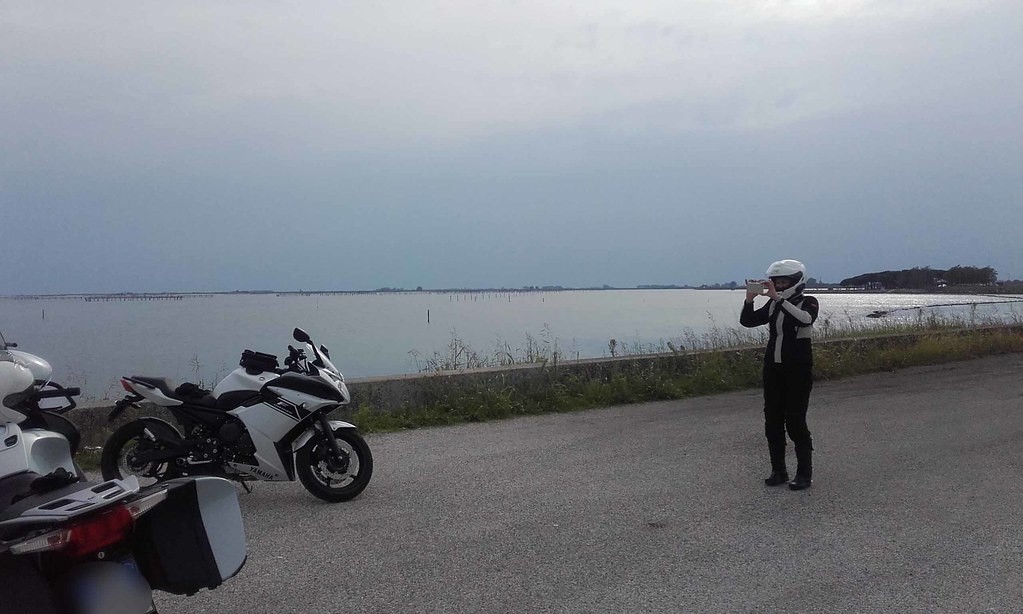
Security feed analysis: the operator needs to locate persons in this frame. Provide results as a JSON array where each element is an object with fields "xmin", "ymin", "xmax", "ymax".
[{"xmin": 739, "ymin": 259, "xmax": 819, "ymax": 489}]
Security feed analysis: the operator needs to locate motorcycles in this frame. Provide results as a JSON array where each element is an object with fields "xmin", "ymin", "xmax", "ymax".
[
  {"xmin": 0, "ymin": 332, "xmax": 248, "ymax": 614},
  {"xmin": 102, "ymin": 327, "xmax": 373, "ymax": 504}
]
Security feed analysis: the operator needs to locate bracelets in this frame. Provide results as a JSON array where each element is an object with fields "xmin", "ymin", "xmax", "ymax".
[{"xmin": 776, "ymin": 296, "xmax": 782, "ymax": 303}]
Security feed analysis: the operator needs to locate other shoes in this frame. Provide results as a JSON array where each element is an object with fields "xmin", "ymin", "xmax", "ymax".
[
  {"xmin": 789, "ymin": 475, "xmax": 811, "ymax": 489},
  {"xmin": 765, "ymin": 472, "xmax": 789, "ymax": 485}
]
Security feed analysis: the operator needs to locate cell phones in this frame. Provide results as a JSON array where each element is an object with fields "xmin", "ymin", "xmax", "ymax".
[{"xmin": 746, "ymin": 281, "xmax": 763, "ymax": 293}]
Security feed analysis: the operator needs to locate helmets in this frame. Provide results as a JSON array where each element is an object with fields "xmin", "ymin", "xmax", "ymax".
[
  {"xmin": 766, "ymin": 259, "xmax": 806, "ymax": 300},
  {"xmin": 0, "ymin": 349, "xmax": 52, "ymax": 427}
]
